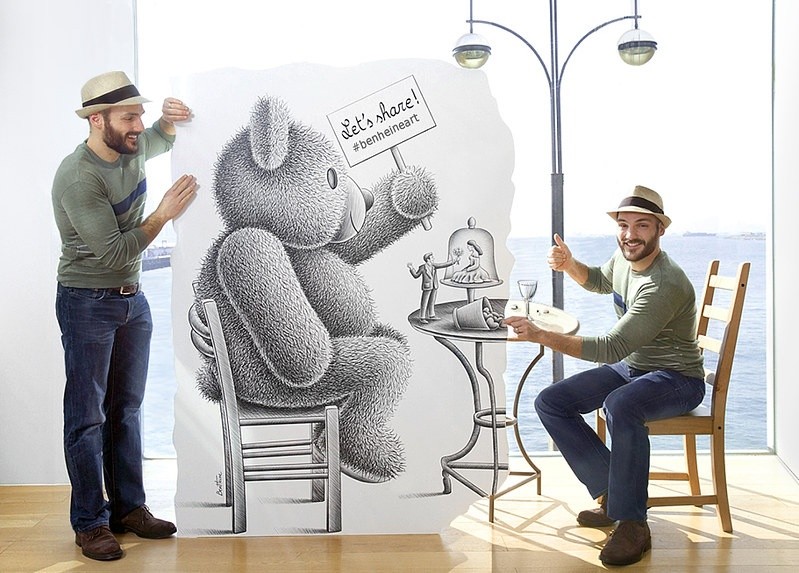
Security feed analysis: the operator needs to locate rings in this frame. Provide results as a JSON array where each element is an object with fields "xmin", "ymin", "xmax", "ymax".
[
  {"xmin": 169, "ymin": 102, "xmax": 172, "ymax": 109},
  {"xmin": 516, "ymin": 327, "xmax": 519, "ymax": 334}
]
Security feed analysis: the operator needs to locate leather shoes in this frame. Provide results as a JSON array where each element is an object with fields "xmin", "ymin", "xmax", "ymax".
[
  {"xmin": 577, "ymin": 493, "xmax": 620, "ymax": 528},
  {"xmin": 74, "ymin": 525, "xmax": 123, "ymax": 560},
  {"xmin": 109, "ymin": 504, "xmax": 177, "ymax": 539},
  {"xmin": 598, "ymin": 518, "xmax": 652, "ymax": 564}
]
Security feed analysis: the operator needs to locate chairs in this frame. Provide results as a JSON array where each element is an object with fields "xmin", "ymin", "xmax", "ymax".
[{"xmin": 597, "ymin": 256, "xmax": 752, "ymax": 531}]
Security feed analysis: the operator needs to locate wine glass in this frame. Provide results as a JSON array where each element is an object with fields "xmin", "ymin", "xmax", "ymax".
[{"xmin": 517, "ymin": 279, "xmax": 538, "ymax": 321}]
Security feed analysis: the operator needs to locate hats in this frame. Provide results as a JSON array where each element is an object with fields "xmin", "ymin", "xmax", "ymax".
[
  {"xmin": 606, "ymin": 186, "xmax": 672, "ymax": 228},
  {"xmin": 75, "ymin": 70, "xmax": 153, "ymax": 119}
]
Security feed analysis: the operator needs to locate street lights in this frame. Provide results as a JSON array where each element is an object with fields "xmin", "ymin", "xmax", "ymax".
[{"xmin": 452, "ymin": 0, "xmax": 659, "ymax": 453}]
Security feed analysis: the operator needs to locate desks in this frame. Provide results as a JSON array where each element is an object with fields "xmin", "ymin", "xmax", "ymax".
[{"xmin": 490, "ymin": 300, "xmax": 579, "ymax": 524}]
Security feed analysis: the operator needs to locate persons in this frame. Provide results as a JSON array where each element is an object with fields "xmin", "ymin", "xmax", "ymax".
[
  {"xmin": 501, "ymin": 184, "xmax": 706, "ymax": 566},
  {"xmin": 51, "ymin": 71, "xmax": 197, "ymax": 561}
]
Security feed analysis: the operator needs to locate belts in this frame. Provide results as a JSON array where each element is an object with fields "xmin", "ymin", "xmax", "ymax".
[{"xmin": 88, "ymin": 284, "xmax": 140, "ymax": 294}]
